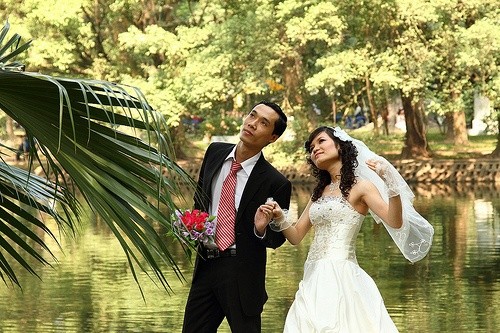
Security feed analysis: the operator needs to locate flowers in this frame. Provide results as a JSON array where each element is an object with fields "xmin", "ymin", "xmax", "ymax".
[{"xmin": 165, "ymin": 208, "xmax": 217, "ymax": 252}]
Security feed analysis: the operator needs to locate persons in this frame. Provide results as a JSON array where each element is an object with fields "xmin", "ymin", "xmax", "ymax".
[
  {"xmin": 265, "ymin": 126, "xmax": 404, "ymax": 333},
  {"xmin": 179, "ymin": 99, "xmax": 293, "ymax": 333}
]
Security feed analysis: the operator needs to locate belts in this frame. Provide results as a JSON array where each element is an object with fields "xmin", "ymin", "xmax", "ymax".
[{"xmin": 198, "ymin": 244, "xmax": 237, "ymax": 259}]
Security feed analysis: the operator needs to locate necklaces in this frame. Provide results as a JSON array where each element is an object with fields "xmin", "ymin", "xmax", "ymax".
[{"xmin": 330, "ymin": 179, "xmax": 343, "ymax": 194}]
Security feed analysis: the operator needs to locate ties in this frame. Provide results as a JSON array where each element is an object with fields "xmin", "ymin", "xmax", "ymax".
[{"xmin": 214, "ymin": 160, "xmax": 242, "ymax": 250}]
